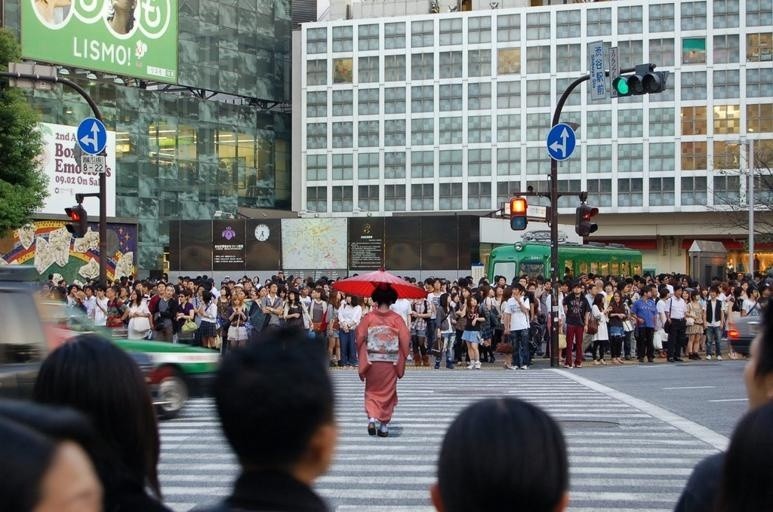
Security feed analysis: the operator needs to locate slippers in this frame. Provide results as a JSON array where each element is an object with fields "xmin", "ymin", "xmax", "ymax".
[
  {"xmin": 368, "ymin": 422, "xmax": 376, "ymax": 435},
  {"xmin": 377, "ymin": 429, "xmax": 387, "ymax": 437}
]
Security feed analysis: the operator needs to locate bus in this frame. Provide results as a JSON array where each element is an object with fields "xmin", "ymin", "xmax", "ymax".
[{"xmin": 483, "ymin": 229, "xmax": 644, "ymax": 283}]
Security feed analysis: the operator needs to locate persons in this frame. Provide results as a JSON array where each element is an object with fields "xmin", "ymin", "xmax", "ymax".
[
  {"xmin": 184, "ymin": 324, "xmax": 338, "ymax": 510},
  {"xmin": 557, "ymin": 272, "xmax": 708, "ymax": 368},
  {"xmin": 390, "ymin": 275, "xmax": 564, "ymax": 369},
  {"xmin": 0, "ymin": 395, "xmax": 107, "ymax": 510},
  {"xmin": 428, "ymin": 395, "xmax": 570, "ymax": 512},
  {"xmin": 354, "ymin": 286, "xmax": 410, "ymax": 437},
  {"xmin": 216, "ymin": 274, "xmax": 371, "ymax": 367},
  {"xmin": 33, "ymin": 333, "xmax": 172, "ymax": 511},
  {"xmin": 703, "ymin": 272, "xmax": 773, "ymax": 360},
  {"xmin": 40, "ymin": 274, "xmax": 219, "ymax": 348},
  {"xmin": 674, "ymin": 292, "xmax": 770, "ymax": 511},
  {"xmin": 717, "ymin": 403, "xmax": 771, "ymax": 510}
]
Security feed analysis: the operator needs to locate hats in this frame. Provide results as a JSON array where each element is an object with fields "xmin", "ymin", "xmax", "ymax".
[
  {"xmin": 286, "ymin": 287, "xmax": 301, "ymax": 295},
  {"xmin": 233, "ymin": 283, "xmax": 243, "ymax": 288}
]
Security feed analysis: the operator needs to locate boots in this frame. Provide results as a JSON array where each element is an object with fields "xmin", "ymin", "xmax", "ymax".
[
  {"xmin": 422, "ymin": 355, "xmax": 430, "ymax": 366},
  {"xmin": 480, "ymin": 347, "xmax": 488, "ymax": 362},
  {"xmin": 435, "ymin": 362, "xmax": 439, "ymax": 369},
  {"xmin": 413, "ymin": 353, "xmax": 421, "ymax": 366},
  {"xmin": 446, "ymin": 360, "xmax": 453, "ymax": 369},
  {"xmin": 486, "ymin": 346, "xmax": 495, "ymax": 363}
]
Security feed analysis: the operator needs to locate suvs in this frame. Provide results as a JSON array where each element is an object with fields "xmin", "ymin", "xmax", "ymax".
[{"xmin": 0, "ymin": 262, "xmax": 172, "ymax": 422}]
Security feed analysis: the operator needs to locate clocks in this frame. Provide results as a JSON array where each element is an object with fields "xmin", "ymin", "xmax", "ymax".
[{"xmin": 253, "ymin": 224, "xmax": 270, "ymax": 241}]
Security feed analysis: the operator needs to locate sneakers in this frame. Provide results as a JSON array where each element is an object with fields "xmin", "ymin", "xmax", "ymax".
[
  {"xmin": 716, "ymin": 355, "xmax": 722, "ymax": 361},
  {"xmin": 474, "ymin": 361, "xmax": 481, "ymax": 369},
  {"xmin": 467, "ymin": 362, "xmax": 474, "ymax": 369},
  {"xmin": 705, "ymin": 354, "xmax": 711, "ymax": 360},
  {"xmin": 520, "ymin": 365, "xmax": 527, "ymax": 370}
]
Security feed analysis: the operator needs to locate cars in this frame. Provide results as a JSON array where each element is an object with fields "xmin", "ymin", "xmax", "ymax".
[
  {"xmin": 39, "ymin": 296, "xmax": 222, "ymax": 420},
  {"xmin": 722, "ymin": 302, "xmax": 764, "ymax": 359}
]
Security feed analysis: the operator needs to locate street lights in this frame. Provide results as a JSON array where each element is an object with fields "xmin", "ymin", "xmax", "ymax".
[{"xmin": 736, "ymin": 136, "xmax": 754, "ymax": 283}]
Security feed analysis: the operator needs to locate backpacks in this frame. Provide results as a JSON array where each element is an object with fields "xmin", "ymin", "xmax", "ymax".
[{"xmin": 320, "ymin": 310, "xmax": 328, "ymax": 332}]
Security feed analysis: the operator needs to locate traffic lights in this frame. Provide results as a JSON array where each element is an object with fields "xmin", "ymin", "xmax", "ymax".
[
  {"xmin": 575, "ymin": 204, "xmax": 598, "ymax": 238},
  {"xmin": 61, "ymin": 205, "xmax": 89, "ymax": 239},
  {"xmin": 509, "ymin": 197, "xmax": 528, "ymax": 232},
  {"xmin": 610, "ymin": 68, "xmax": 666, "ymax": 97}
]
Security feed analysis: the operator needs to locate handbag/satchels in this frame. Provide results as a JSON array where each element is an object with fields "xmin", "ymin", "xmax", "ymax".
[
  {"xmin": 133, "ymin": 317, "xmax": 150, "ymax": 332},
  {"xmin": 621, "ymin": 318, "xmax": 635, "ymax": 332},
  {"xmin": 430, "ymin": 335, "xmax": 444, "ymax": 357},
  {"xmin": 685, "ymin": 303, "xmax": 695, "ymax": 326},
  {"xmin": 585, "ymin": 311, "xmax": 598, "ymax": 334},
  {"xmin": 558, "ymin": 324, "xmax": 567, "ymax": 350},
  {"xmin": 664, "ymin": 319, "xmax": 671, "ymax": 333},
  {"xmin": 181, "ymin": 316, "xmax": 197, "ymax": 333},
  {"xmin": 250, "ymin": 298, "xmax": 271, "ymax": 332}
]
